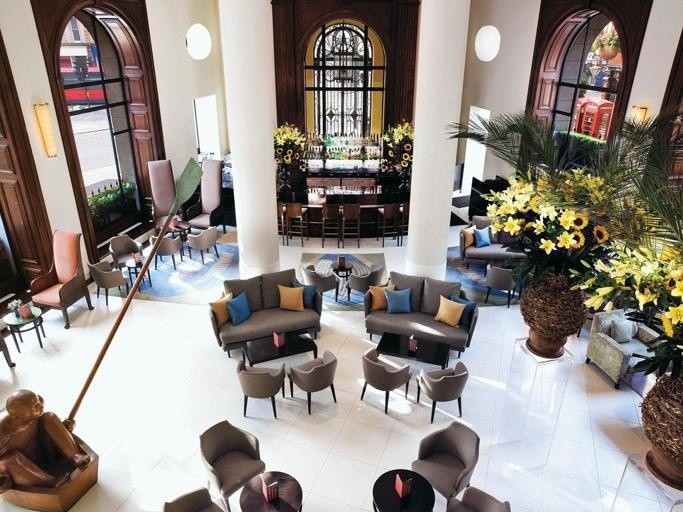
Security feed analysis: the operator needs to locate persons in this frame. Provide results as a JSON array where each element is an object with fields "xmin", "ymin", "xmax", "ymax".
[{"xmin": 0, "ymin": 389, "xmax": 90, "ymax": 494}]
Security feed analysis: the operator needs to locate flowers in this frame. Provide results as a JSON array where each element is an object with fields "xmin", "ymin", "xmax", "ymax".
[
  {"xmin": 273, "ymin": 123, "xmax": 306, "ymax": 167},
  {"xmin": 440, "ymin": 107, "xmax": 656, "ymax": 288},
  {"xmin": 380, "ymin": 121, "xmax": 416, "ymax": 173},
  {"xmin": 573, "ymin": 105, "xmax": 683, "ymax": 378}
]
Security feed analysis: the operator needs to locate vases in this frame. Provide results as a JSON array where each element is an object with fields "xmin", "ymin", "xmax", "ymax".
[
  {"xmin": 638, "ymin": 360, "xmax": 681, "ymax": 482},
  {"xmin": 397, "ymin": 165, "xmax": 410, "ymax": 191},
  {"xmin": 278, "ymin": 167, "xmax": 291, "ymax": 191},
  {"xmin": 514, "ymin": 269, "xmax": 591, "ymax": 360}
]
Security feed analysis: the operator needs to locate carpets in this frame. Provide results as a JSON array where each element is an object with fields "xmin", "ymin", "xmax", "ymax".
[{"xmin": 299, "ymin": 252, "xmax": 389, "ymax": 311}]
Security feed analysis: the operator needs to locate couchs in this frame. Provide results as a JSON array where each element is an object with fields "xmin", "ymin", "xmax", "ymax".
[
  {"xmin": 209, "ymin": 269, "xmax": 321, "ymax": 359},
  {"xmin": 364, "ymin": 271, "xmax": 480, "ymax": 358},
  {"xmin": 584, "ymin": 307, "xmax": 661, "ymax": 391}
]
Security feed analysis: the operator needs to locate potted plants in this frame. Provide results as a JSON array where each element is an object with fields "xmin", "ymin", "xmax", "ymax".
[{"xmin": 592, "ymin": 28, "xmax": 621, "ymax": 66}]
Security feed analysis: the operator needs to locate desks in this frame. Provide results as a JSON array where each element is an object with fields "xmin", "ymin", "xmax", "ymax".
[
  {"xmin": 241, "ymin": 328, "xmax": 318, "ymax": 367},
  {"xmin": 2, "ymin": 306, "xmax": 45, "ymax": 352},
  {"xmin": 377, "ymin": 332, "xmax": 450, "ymax": 370}
]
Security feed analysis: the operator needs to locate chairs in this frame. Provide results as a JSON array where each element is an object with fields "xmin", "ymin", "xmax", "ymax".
[
  {"xmin": 236, "ymin": 359, "xmax": 285, "ymax": 420},
  {"xmin": 447, "ymin": 487, "xmax": 512, "ymax": 512},
  {"xmin": 22, "ymin": 153, "xmax": 224, "ymax": 328},
  {"xmin": 360, "ymin": 348, "xmax": 413, "ymax": 415},
  {"xmin": 411, "ymin": 422, "xmax": 481, "ymax": 504},
  {"xmin": 301, "ymin": 264, "xmax": 340, "ymax": 302},
  {"xmin": 199, "ymin": 420, "xmax": 265, "ymax": 512},
  {"xmin": 162, "ymin": 487, "xmax": 224, "ymax": 512},
  {"xmin": 415, "ymin": 361, "xmax": 469, "ymax": 424},
  {"xmin": 287, "ymin": 351, "xmax": 337, "ymax": 416},
  {"xmin": 348, "ymin": 264, "xmax": 385, "ymax": 302}
]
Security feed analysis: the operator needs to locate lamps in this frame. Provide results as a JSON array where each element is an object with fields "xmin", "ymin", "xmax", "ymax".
[{"xmin": 29, "ymin": 99, "xmax": 61, "ymax": 158}]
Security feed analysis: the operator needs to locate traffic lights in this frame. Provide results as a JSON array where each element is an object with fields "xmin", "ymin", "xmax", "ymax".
[{"xmin": 70, "ymin": 56, "xmax": 83, "ymax": 75}]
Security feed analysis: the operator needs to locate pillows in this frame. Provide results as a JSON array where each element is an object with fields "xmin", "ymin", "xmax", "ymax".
[
  {"xmin": 384, "ymin": 288, "xmax": 411, "ymax": 313},
  {"xmin": 227, "ymin": 289, "xmax": 251, "ymax": 326},
  {"xmin": 433, "ymin": 295, "xmax": 467, "ymax": 328},
  {"xmin": 610, "ymin": 319, "xmax": 633, "ymax": 345},
  {"xmin": 368, "ymin": 283, "xmax": 395, "ymax": 312},
  {"xmin": 209, "ymin": 292, "xmax": 233, "ymax": 327},
  {"xmin": 292, "ymin": 279, "xmax": 316, "ymax": 310},
  {"xmin": 277, "ymin": 284, "xmax": 304, "ymax": 312},
  {"xmin": 450, "ymin": 294, "xmax": 478, "ymax": 330}
]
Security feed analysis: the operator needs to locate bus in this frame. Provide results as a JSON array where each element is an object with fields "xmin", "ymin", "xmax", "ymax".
[{"xmin": 59, "ymin": 68, "xmax": 105, "ymax": 105}]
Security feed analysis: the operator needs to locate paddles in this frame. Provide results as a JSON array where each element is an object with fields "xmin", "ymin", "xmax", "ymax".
[{"xmin": 68, "ymin": 157, "xmax": 203, "ymax": 422}]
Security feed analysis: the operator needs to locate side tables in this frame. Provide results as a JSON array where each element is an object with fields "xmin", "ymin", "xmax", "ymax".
[
  {"xmin": 239, "ymin": 470, "xmax": 303, "ymax": 512},
  {"xmin": 331, "ymin": 262, "xmax": 353, "ymax": 303},
  {"xmin": 372, "ymin": 469, "xmax": 436, "ymax": 512}
]
